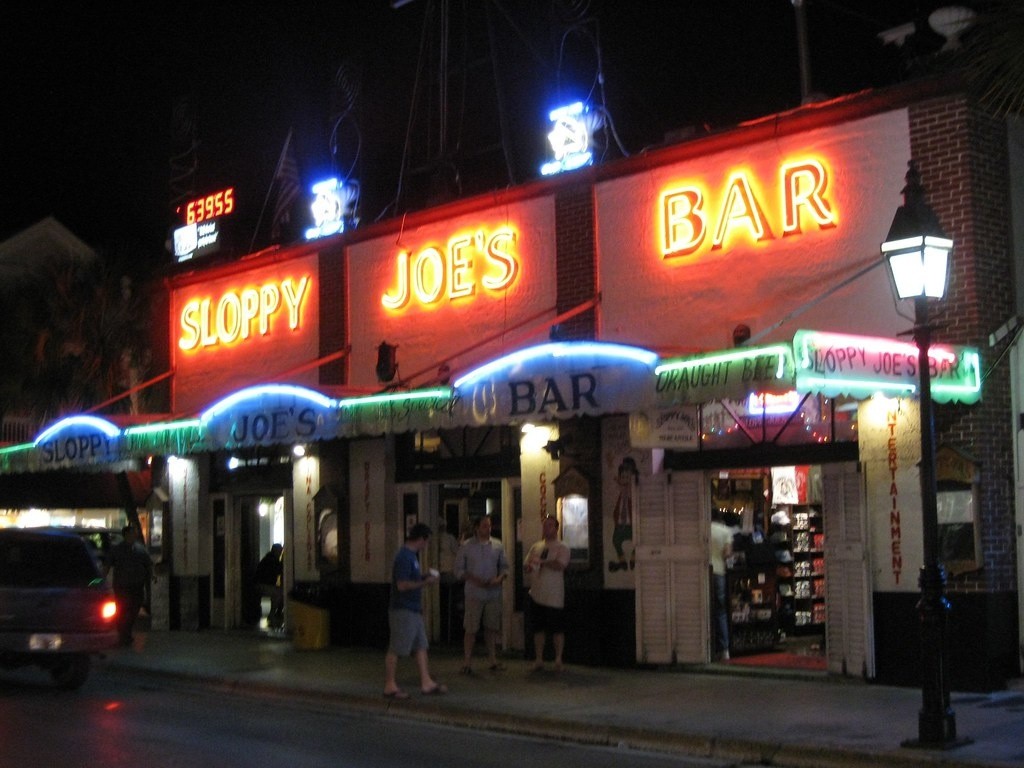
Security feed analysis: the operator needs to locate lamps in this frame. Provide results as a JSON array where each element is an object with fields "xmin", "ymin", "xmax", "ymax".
[
  {"xmin": 519, "ymin": 423, "xmax": 572, "ymax": 460},
  {"xmin": 375, "ymin": 341, "xmax": 399, "ymax": 382}
]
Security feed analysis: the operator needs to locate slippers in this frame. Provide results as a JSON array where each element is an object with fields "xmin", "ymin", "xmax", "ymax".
[
  {"xmin": 421, "ymin": 682, "xmax": 449, "ymax": 694},
  {"xmin": 383, "ymin": 689, "xmax": 412, "ymax": 699},
  {"xmin": 487, "ymin": 661, "xmax": 507, "ymax": 670}
]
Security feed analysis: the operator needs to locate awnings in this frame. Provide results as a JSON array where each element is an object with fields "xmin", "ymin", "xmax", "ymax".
[{"xmin": 0, "ymin": 329, "xmax": 979, "ymax": 481}]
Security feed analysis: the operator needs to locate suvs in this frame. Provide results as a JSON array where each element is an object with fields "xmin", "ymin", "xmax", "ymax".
[{"xmin": 0, "ymin": 527, "xmax": 121, "ymax": 703}]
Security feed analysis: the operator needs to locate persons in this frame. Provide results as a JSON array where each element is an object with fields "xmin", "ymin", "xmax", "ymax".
[
  {"xmin": 709, "ymin": 507, "xmax": 733, "ymax": 660},
  {"xmin": 438, "ymin": 518, "xmax": 466, "ymax": 616},
  {"xmin": 102, "ymin": 526, "xmax": 151, "ymax": 644},
  {"xmin": 256, "ymin": 544, "xmax": 283, "ymax": 632},
  {"xmin": 523, "ymin": 517, "xmax": 570, "ymax": 673},
  {"xmin": 454, "ymin": 515, "xmax": 509, "ymax": 676},
  {"xmin": 383, "ymin": 524, "xmax": 448, "ymax": 699},
  {"xmin": 104, "ymin": 348, "xmax": 140, "ymax": 415}
]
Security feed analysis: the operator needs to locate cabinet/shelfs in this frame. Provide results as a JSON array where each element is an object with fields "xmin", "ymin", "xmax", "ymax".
[{"xmin": 771, "ymin": 502, "xmax": 827, "ymax": 635}]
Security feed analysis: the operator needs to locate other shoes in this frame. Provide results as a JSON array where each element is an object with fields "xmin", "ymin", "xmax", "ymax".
[
  {"xmin": 268, "ymin": 608, "xmax": 283, "ymax": 618},
  {"xmin": 551, "ymin": 664, "xmax": 570, "ymax": 672},
  {"xmin": 534, "ymin": 665, "xmax": 550, "ymax": 673}
]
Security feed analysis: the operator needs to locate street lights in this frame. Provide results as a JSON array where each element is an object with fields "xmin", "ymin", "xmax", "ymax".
[{"xmin": 879, "ymin": 159, "xmax": 973, "ymax": 750}]
